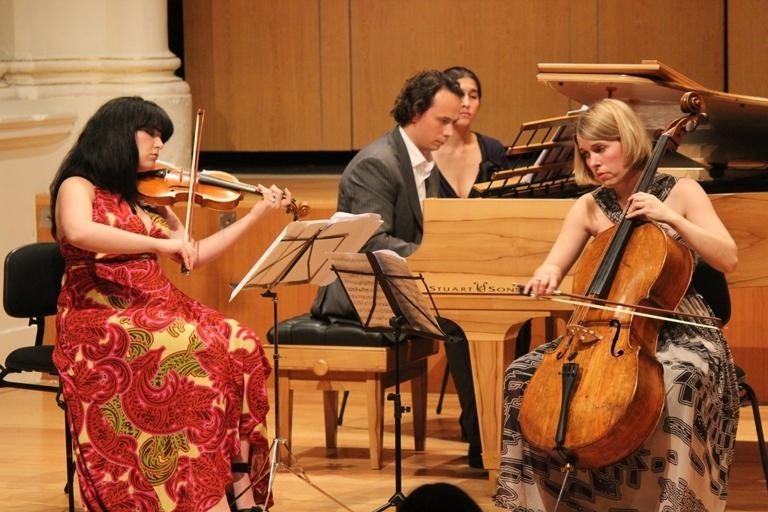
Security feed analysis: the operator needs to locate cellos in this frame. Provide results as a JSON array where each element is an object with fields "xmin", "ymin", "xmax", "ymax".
[{"xmin": 518, "ymin": 92, "xmax": 707, "ymax": 471}]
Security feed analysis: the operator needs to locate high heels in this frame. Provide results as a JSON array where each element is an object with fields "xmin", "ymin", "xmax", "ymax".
[{"xmin": 224, "ymin": 463, "xmax": 269, "ymax": 512}]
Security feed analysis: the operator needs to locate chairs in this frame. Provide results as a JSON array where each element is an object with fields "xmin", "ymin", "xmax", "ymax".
[
  {"xmin": 0, "ymin": 241, "xmax": 79, "ymax": 512},
  {"xmin": 683, "ymin": 256, "xmax": 766, "ymax": 488}
]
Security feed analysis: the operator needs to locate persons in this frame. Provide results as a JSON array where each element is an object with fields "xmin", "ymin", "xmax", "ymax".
[
  {"xmin": 423, "ymin": 66, "xmax": 515, "ymax": 199},
  {"xmin": 50, "ymin": 96, "xmax": 295, "ymax": 511},
  {"xmin": 312, "ymin": 69, "xmax": 478, "ymax": 468},
  {"xmin": 493, "ymin": 98, "xmax": 739, "ymax": 512}
]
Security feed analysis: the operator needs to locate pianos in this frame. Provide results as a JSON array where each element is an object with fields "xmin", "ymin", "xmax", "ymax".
[{"xmin": 405, "ymin": 61, "xmax": 768, "ymax": 497}]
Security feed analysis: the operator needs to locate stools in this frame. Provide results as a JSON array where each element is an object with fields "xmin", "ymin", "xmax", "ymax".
[{"xmin": 261, "ymin": 306, "xmax": 440, "ymax": 474}]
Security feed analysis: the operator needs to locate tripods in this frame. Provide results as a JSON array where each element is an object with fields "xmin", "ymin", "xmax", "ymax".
[{"xmin": 229, "ymin": 303, "xmax": 353, "ymax": 510}]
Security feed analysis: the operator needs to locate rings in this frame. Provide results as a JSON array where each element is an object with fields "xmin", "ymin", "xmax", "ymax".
[{"xmin": 535, "ymin": 278, "xmax": 542, "ymax": 282}]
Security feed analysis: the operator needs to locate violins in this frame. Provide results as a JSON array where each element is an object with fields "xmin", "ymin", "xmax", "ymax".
[{"xmin": 136, "ymin": 161, "xmax": 310, "ymax": 221}]
